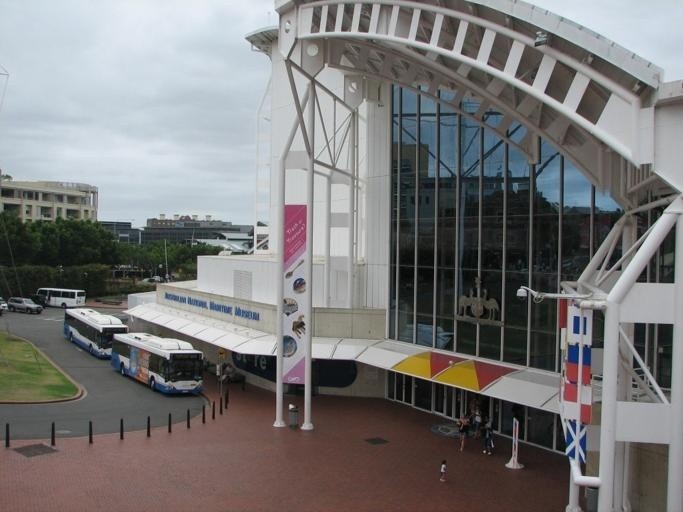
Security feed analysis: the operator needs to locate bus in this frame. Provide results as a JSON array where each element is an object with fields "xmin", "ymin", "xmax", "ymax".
[
  {"xmin": 36, "ymin": 288, "xmax": 87, "ymax": 309},
  {"xmin": 63, "ymin": 305, "xmax": 130, "ymax": 362},
  {"xmin": 109, "ymin": 329, "xmax": 206, "ymax": 398}
]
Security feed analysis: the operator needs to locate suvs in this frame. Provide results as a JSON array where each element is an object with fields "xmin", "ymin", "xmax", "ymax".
[
  {"xmin": 0, "ymin": 297, "xmax": 8, "ymax": 316},
  {"xmin": 7, "ymin": 297, "xmax": 43, "ymax": 314},
  {"xmin": 30, "ymin": 295, "xmax": 46, "ymax": 309}
]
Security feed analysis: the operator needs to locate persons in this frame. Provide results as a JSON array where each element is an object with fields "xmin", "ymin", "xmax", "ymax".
[
  {"xmin": 439, "ymin": 460, "xmax": 447, "ymax": 482},
  {"xmin": 455, "ymin": 392, "xmax": 496, "ymax": 456}
]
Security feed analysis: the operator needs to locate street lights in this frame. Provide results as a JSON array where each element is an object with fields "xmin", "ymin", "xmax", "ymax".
[
  {"xmin": 84, "ymin": 271, "xmax": 88, "ymax": 302},
  {"xmin": 158, "ymin": 263, "xmax": 163, "ymax": 282},
  {"xmin": 59, "ymin": 265, "xmax": 64, "ymax": 288}
]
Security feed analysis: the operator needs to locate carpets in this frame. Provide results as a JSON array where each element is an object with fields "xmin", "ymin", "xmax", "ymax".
[{"xmin": 430, "ymin": 422, "xmax": 475, "ymax": 439}]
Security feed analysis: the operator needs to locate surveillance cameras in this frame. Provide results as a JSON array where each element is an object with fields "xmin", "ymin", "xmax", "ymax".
[{"xmin": 516, "ymin": 288, "xmax": 527, "ymax": 301}]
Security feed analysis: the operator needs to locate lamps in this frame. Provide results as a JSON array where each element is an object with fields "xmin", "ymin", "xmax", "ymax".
[{"xmin": 534, "ymin": 32, "xmax": 548, "ymax": 47}]
